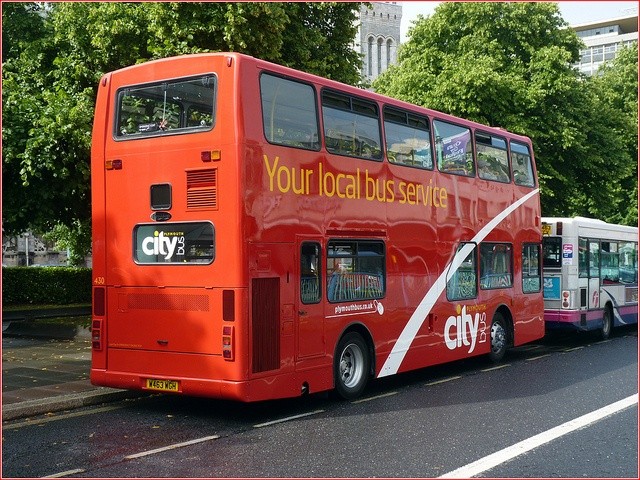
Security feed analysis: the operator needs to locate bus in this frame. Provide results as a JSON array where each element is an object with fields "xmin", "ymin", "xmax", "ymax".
[
  {"xmin": 91, "ymin": 52, "xmax": 545, "ymax": 402},
  {"xmin": 541, "ymin": 216, "xmax": 639, "ymax": 339}
]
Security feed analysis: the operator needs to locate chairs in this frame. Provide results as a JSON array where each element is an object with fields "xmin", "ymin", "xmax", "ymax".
[
  {"xmin": 388, "ymin": 156, "xmax": 405, "ymax": 165},
  {"xmin": 446, "ymin": 168, "xmax": 467, "ymax": 175}
]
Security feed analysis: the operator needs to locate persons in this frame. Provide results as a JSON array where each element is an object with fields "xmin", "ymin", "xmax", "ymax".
[{"xmin": 477, "ymin": 161, "xmax": 497, "ymax": 181}]
